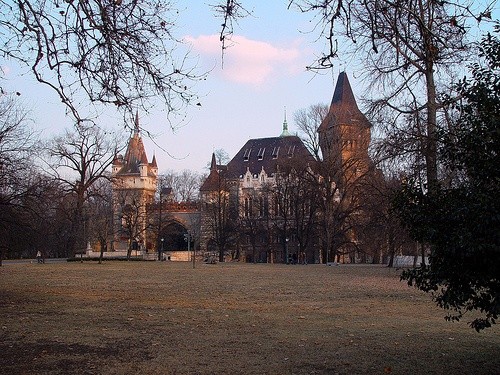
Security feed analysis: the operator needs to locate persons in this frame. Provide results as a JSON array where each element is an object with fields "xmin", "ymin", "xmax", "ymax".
[
  {"xmin": 288, "ymin": 251, "xmax": 307, "ymax": 265},
  {"xmin": 36, "ymin": 249, "xmax": 42, "ymax": 263}
]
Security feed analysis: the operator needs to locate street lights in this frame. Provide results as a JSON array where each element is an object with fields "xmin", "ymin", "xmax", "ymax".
[{"xmin": 157, "ymin": 177, "xmax": 169, "ymax": 256}]
